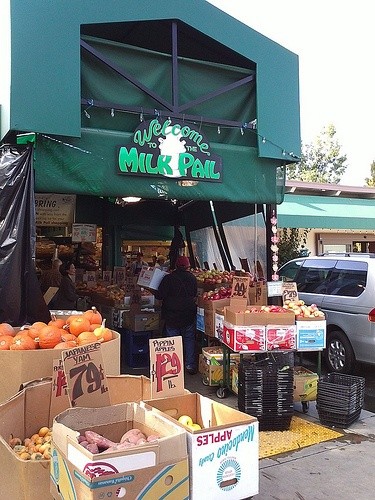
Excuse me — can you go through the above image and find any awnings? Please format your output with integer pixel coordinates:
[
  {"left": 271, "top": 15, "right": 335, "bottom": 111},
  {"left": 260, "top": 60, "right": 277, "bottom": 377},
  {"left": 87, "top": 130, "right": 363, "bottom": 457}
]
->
[{"left": 223, "top": 194, "right": 375, "bottom": 230}]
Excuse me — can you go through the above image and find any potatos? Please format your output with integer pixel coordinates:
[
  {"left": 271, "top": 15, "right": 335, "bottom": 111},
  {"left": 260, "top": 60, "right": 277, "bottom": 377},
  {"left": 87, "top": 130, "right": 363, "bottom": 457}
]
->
[{"left": 77, "top": 428, "right": 159, "bottom": 454}]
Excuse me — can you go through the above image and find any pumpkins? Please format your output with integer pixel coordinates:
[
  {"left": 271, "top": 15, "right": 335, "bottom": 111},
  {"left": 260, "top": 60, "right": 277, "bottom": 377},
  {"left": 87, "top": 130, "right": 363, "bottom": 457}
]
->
[{"left": 0, "top": 306, "right": 112, "bottom": 351}]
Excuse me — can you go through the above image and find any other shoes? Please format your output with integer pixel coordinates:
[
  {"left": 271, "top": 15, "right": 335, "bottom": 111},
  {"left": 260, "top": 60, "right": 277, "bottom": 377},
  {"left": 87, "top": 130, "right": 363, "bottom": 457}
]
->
[{"left": 186, "top": 369, "right": 195, "bottom": 375}]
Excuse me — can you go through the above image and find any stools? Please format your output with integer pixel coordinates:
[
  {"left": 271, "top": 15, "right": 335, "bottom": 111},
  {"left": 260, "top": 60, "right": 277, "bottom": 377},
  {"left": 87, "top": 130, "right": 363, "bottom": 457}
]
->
[{"left": 125, "top": 329, "right": 153, "bottom": 369}]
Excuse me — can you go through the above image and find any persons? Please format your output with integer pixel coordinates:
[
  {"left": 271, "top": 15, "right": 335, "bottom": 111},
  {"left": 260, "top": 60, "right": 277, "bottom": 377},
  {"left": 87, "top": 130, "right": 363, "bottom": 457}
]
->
[
  {"left": 150, "top": 255, "right": 164, "bottom": 267},
  {"left": 57, "top": 262, "right": 78, "bottom": 310},
  {"left": 150, "top": 256, "right": 198, "bottom": 373},
  {"left": 131, "top": 252, "right": 148, "bottom": 274},
  {"left": 39, "top": 258, "right": 63, "bottom": 309}
]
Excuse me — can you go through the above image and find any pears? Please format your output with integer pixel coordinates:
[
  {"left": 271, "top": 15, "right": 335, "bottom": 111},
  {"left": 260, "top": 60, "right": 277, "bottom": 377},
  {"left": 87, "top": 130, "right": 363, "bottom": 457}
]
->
[
  {"left": 111, "top": 291, "right": 125, "bottom": 301},
  {"left": 10, "top": 425, "right": 49, "bottom": 460}
]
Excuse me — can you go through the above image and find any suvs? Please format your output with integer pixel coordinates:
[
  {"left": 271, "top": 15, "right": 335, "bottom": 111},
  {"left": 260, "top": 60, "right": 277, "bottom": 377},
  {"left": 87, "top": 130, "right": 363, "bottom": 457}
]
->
[{"left": 275, "top": 252, "right": 375, "bottom": 374}]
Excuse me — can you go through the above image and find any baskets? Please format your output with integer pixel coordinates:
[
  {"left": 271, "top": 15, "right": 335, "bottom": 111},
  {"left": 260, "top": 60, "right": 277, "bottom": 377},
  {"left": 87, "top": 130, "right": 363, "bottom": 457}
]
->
[
  {"left": 237, "top": 363, "right": 294, "bottom": 431},
  {"left": 316, "top": 372, "right": 365, "bottom": 429}
]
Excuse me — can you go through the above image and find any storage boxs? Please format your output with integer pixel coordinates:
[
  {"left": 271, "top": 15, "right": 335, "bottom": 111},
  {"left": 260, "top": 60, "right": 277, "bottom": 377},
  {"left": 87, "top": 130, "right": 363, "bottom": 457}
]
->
[
  {"left": 0, "top": 310, "right": 260, "bottom": 500},
  {"left": 78, "top": 291, "right": 163, "bottom": 332},
  {"left": 197, "top": 281, "right": 327, "bottom": 401},
  {"left": 136, "top": 265, "right": 170, "bottom": 290}
]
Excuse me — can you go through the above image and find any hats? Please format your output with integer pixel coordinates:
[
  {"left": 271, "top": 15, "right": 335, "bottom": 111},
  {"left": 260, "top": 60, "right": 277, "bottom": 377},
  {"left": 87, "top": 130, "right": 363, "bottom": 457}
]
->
[
  {"left": 176, "top": 256, "right": 190, "bottom": 268},
  {"left": 157, "top": 255, "right": 165, "bottom": 260},
  {"left": 137, "top": 252, "right": 143, "bottom": 257}
]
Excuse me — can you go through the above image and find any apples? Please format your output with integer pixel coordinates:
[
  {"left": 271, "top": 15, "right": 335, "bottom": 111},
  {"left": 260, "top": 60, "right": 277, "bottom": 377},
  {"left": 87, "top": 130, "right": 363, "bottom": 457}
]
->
[
  {"left": 192, "top": 269, "right": 234, "bottom": 300},
  {"left": 178, "top": 415, "right": 202, "bottom": 432},
  {"left": 239, "top": 300, "right": 324, "bottom": 317}
]
